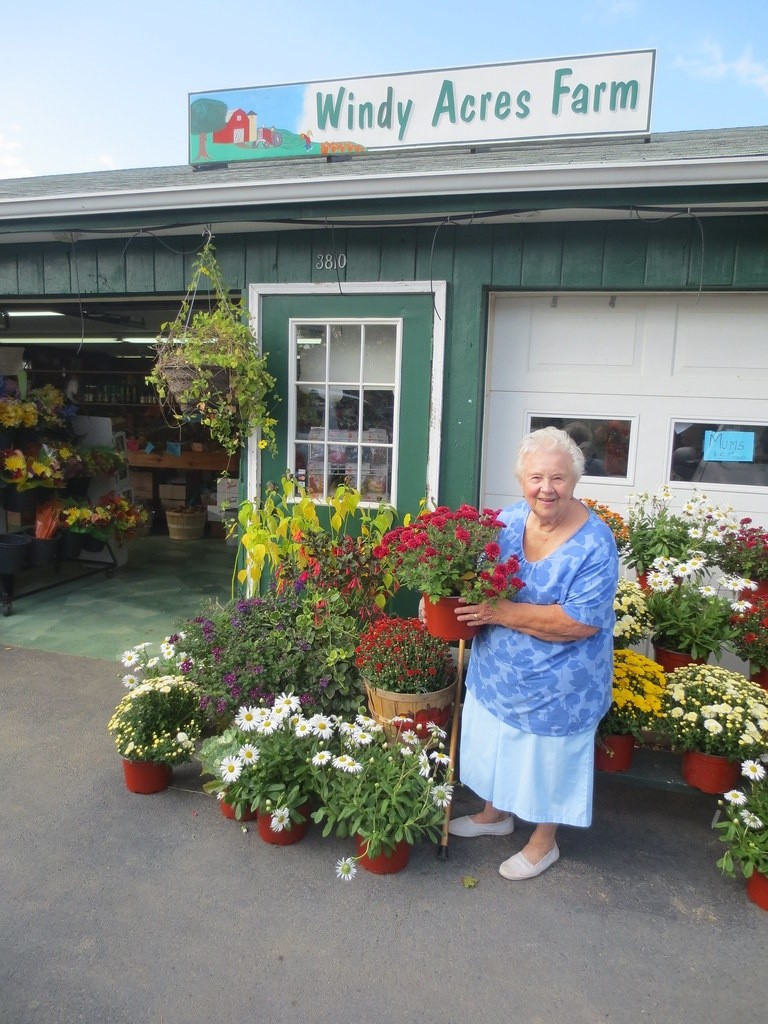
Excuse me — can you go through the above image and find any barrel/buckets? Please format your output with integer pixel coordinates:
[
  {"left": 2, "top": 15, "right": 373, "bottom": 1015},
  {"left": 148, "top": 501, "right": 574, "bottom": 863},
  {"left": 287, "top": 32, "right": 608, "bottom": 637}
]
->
[
  {"left": 0, "top": 534, "right": 33, "bottom": 574},
  {"left": 167, "top": 509, "right": 207, "bottom": 540},
  {"left": 26, "top": 530, "right": 60, "bottom": 566},
  {"left": 367, "top": 669, "right": 461, "bottom": 752}
]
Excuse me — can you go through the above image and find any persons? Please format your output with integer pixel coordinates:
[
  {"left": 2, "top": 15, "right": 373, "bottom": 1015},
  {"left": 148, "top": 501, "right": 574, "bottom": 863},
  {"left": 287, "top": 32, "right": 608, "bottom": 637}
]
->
[{"left": 422, "top": 427, "right": 618, "bottom": 881}]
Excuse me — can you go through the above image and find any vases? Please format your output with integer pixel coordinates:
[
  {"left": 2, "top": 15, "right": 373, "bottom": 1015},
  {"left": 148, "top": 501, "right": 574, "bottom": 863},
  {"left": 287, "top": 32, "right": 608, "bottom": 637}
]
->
[
  {"left": 2, "top": 481, "right": 38, "bottom": 512},
  {"left": 253, "top": 803, "right": 310, "bottom": 847},
  {"left": 123, "top": 758, "right": 173, "bottom": 795},
  {"left": 596, "top": 734, "right": 636, "bottom": 771},
  {"left": 220, "top": 797, "right": 257, "bottom": 822},
  {"left": 355, "top": 833, "right": 408, "bottom": 876},
  {"left": 58, "top": 477, "right": 92, "bottom": 502},
  {"left": 747, "top": 867, "right": 768, "bottom": 911},
  {"left": 748, "top": 659, "right": 768, "bottom": 692},
  {"left": 423, "top": 591, "right": 482, "bottom": 641},
  {"left": 650, "top": 635, "right": 707, "bottom": 674},
  {"left": 167, "top": 509, "right": 207, "bottom": 539},
  {"left": 0, "top": 534, "right": 106, "bottom": 575},
  {"left": 682, "top": 749, "right": 742, "bottom": 794}
]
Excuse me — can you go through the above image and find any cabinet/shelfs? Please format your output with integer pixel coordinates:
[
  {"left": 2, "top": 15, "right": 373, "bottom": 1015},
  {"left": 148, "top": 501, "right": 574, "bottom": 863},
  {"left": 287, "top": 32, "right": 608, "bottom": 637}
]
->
[{"left": 17, "top": 362, "right": 239, "bottom": 472}]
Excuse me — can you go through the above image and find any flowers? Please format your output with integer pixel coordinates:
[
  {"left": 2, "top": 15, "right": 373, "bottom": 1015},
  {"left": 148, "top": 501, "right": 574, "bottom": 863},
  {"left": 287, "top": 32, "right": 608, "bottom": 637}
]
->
[
  {"left": 106, "top": 471, "right": 768, "bottom": 879},
  {"left": 0, "top": 383, "right": 149, "bottom": 540}
]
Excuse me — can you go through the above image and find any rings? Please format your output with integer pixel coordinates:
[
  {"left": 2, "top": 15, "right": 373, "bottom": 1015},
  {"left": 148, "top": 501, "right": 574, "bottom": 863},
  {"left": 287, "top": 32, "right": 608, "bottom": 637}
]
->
[{"left": 475, "top": 613, "right": 480, "bottom": 621}]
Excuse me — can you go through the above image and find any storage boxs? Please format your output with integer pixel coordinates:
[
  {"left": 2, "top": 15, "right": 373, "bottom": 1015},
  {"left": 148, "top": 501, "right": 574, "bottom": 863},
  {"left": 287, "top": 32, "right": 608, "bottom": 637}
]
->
[
  {"left": 158, "top": 484, "right": 187, "bottom": 511},
  {"left": 208, "top": 505, "right": 224, "bottom": 522},
  {"left": 205, "top": 478, "right": 220, "bottom": 510},
  {"left": 216, "top": 477, "right": 238, "bottom": 510},
  {"left": 128, "top": 472, "right": 154, "bottom": 499}
]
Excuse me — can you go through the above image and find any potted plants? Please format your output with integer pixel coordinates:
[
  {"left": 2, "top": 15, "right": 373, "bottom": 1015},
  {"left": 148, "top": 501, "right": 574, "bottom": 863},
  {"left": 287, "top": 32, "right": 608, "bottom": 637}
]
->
[{"left": 145, "top": 232, "right": 281, "bottom": 483}]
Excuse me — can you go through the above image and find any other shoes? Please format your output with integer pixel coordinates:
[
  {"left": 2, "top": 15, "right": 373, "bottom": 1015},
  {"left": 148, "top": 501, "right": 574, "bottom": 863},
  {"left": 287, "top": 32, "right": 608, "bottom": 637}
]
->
[
  {"left": 448, "top": 814, "right": 514, "bottom": 838},
  {"left": 499, "top": 840, "right": 560, "bottom": 880}
]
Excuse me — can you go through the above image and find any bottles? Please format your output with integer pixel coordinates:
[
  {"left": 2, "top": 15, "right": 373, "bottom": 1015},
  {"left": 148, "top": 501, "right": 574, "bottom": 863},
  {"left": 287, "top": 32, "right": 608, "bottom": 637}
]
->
[{"left": 83, "top": 381, "right": 156, "bottom": 404}]
